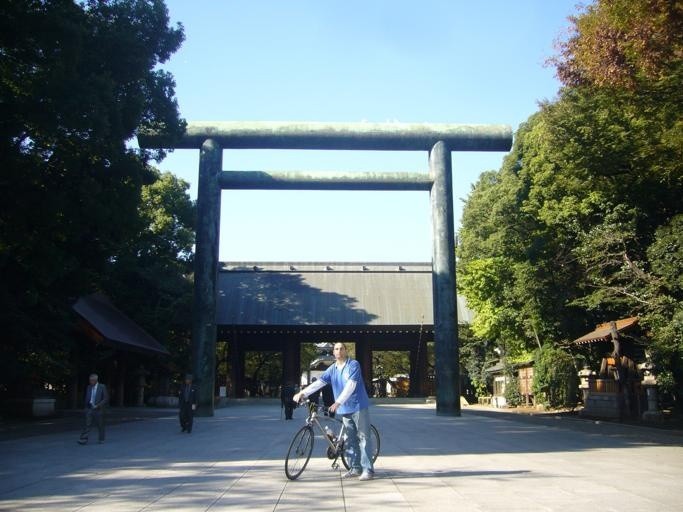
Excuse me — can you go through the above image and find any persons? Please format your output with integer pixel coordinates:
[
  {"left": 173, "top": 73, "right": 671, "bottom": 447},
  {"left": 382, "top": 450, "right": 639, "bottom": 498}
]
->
[
  {"left": 269, "top": 376, "right": 335, "bottom": 421},
  {"left": 291, "top": 341, "right": 375, "bottom": 481},
  {"left": 76, "top": 373, "right": 109, "bottom": 445},
  {"left": 175, "top": 374, "right": 196, "bottom": 435}
]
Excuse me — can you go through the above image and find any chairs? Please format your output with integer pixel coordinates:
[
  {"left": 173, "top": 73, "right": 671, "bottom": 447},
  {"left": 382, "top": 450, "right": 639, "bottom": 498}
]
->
[
  {"left": 339, "top": 468, "right": 362, "bottom": 479},
  {"left": 77, "top": 440, "right": 106, "bottom": 445},
  {"left": 358, "top": 469, "right": 374, "bottom": 480}
]
